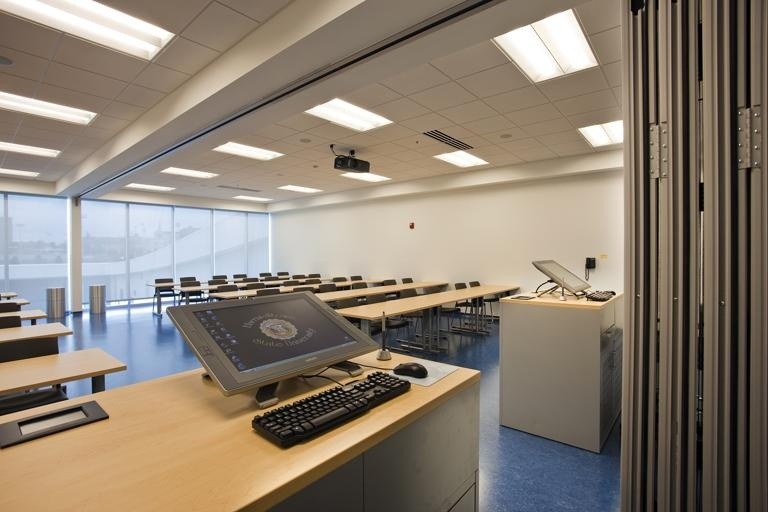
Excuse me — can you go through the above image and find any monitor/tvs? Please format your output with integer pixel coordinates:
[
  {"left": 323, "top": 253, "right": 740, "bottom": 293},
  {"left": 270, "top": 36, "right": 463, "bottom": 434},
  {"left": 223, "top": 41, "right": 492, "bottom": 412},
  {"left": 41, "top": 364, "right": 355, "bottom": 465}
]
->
[
  {"left": 532, "top": 260, "right": 591, "bottom": 301},
  {"left": 166, "top": 291, "right": 381, "bottom": 408}
]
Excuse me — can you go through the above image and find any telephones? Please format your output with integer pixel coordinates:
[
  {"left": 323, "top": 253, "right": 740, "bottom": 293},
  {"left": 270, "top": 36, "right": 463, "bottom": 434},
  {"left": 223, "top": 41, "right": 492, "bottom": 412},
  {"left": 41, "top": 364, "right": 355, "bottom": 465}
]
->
[{"left": 586, "top": 258, "right": 595, "bottom": 268}]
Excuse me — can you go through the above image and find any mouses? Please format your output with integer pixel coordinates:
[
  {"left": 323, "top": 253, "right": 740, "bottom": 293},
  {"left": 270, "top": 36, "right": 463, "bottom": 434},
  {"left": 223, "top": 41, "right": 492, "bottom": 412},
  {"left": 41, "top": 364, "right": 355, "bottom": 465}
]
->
[
  {"left": 393, "top": 362, "right": 428, "bottom": 378},
  {"left": 610, "top": 291, "right": 616, "bottom": 295}
]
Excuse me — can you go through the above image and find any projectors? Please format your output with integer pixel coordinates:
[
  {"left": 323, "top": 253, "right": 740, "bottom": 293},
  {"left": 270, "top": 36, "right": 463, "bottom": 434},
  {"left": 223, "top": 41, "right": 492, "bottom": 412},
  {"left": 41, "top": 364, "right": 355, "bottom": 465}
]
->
[{"left": 334, "top": 157, "right": 370, "bottom": 173}]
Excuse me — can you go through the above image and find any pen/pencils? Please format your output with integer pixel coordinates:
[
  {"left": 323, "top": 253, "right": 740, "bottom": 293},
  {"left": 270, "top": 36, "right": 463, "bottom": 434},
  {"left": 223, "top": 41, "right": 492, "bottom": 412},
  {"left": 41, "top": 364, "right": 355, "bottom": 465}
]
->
[
  {"left": 382, "top": 311, "right": 385, "bottom": 351},
  {"left": 562, "top": 277, "right": 564, "bottom": 296}
]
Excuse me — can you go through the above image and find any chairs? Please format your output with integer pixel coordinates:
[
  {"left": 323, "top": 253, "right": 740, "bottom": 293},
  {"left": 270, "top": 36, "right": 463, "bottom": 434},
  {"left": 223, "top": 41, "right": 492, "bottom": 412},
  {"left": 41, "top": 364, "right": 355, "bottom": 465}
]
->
[
  {"left": 212, "top": 275, "right": 228, "bottom": 280},
  {"left": 177, "top": 277, "right": 196, "bottom": 305},
  {"left": 352, "top": 283, "right": 368, "bottom": 288},
  {"left": 306, "top": 279, "right": 321, "bottom": 284},
  {"left": 450, "top": 283, "right": 486, "bottom": 336},
  {"left": 365, "top": 293, "right": 411, "bottom": 355},
  {"left": 294, "top": 287, "right": 315, "bottom": 294},
  {"left": 181, "top": 281, "right": 205, "bottom": 305},
  {"left": 257, "top": 288, "right": 280, "bottom": 296},
  {"left": 277, "top": 272, "right": 289, "bottom": 276},
  {"left": 397, "top": 288, "right": 440, "bottom": 350},
  {"left": 309, "top": 274, "right": 321, "bottom": 278},
  {"left": 401, "top": 277, "right": 414, "bottom": 283},
  {"left": 259, "top": 273, "right": 271, "bottom": 277},
  {"left": 233, "top": 274, "right": 247, "bottom": 278},
  {"left": 336, "top": 297, "right": 362, "bottom": 331},
  {"left": 247, "top": 283, "right": 264, "bottom": 289},
  {"left": 384, "top": 279, "right": 396, "bottom": 285},
  {"left": 293, "top": 275, "right": 305, "bottom": 278},
  {"left": 152, "top": 279, "right": 176, "bottom": 312},
  {"left": 284, "top": 281, "right": 299, "bottom": 285},
  {"left": 423, "top": 284, "right": 463, "bottom": 339},
  {"left": 319, "top": 284, "right": 336, "bottom": 293},
  {"left": 350, "top": 275, "right": 363, "bottom": 280},
  {"left": 332, "top": 277, "right": 347, "bottom": 283},
  {"left": 243, "top": 278, "right": 258, "bottom": 281},
  {"left": 469, "top": 281, "right": 499, "bottom": 324},
  {"left": 218, "top": 285, "right": 240, "bottom": 301},
  {"left": 265, "top": 277, "right": 279, "bottom": 281},
  {"left": 207, "top": 280, "right": 225, "bottom": 303}
]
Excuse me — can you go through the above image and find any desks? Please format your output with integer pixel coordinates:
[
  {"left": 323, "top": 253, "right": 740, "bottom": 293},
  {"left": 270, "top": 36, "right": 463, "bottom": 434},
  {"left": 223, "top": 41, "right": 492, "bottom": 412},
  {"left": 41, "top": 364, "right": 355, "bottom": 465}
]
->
[
  {"left": 0, "top": 292, "right": 128, "bottom": 420},
  {"left": 313, "top": 281, "right": 448, "bottom": 307},
  {"left": 145, "top": 275, "right": 292, "bottom": 318},
  {"left": 335, "top": 281, "right": 519, "bottom": 358},
  {"left": 209, "top": 280, "right": 355, "bottom": 299}
]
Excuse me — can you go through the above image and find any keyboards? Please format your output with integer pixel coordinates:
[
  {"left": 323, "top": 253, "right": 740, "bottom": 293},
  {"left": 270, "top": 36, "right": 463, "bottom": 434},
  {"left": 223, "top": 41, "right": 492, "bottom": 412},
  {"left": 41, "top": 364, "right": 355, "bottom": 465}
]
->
[
  {"left": 586, "top": 291, "right": 613, "bottom": 301},
  {"left": 252, "top": 371, "right": 411, "bottom": 449}
]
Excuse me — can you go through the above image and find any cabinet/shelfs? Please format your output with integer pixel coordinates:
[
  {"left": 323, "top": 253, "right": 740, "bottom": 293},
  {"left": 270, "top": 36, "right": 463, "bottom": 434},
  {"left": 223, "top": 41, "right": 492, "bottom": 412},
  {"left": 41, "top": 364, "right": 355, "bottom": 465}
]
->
[
  {"left": 0, "top": 338, "right": 483, "bottom": 512},
  {"left": 496, "top": 278, "right": 624, "bottom": 458}
]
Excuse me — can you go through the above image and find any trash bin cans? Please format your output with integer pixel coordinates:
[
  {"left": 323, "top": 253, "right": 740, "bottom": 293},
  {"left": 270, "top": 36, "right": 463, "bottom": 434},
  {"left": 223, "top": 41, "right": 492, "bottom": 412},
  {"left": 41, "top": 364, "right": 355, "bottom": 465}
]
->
[
  {"left": 47, "top": 288, "right": 65, "bottom": 319},
  {"left": 89, "top": 286, "right": 106, "bottom": 314}
]
